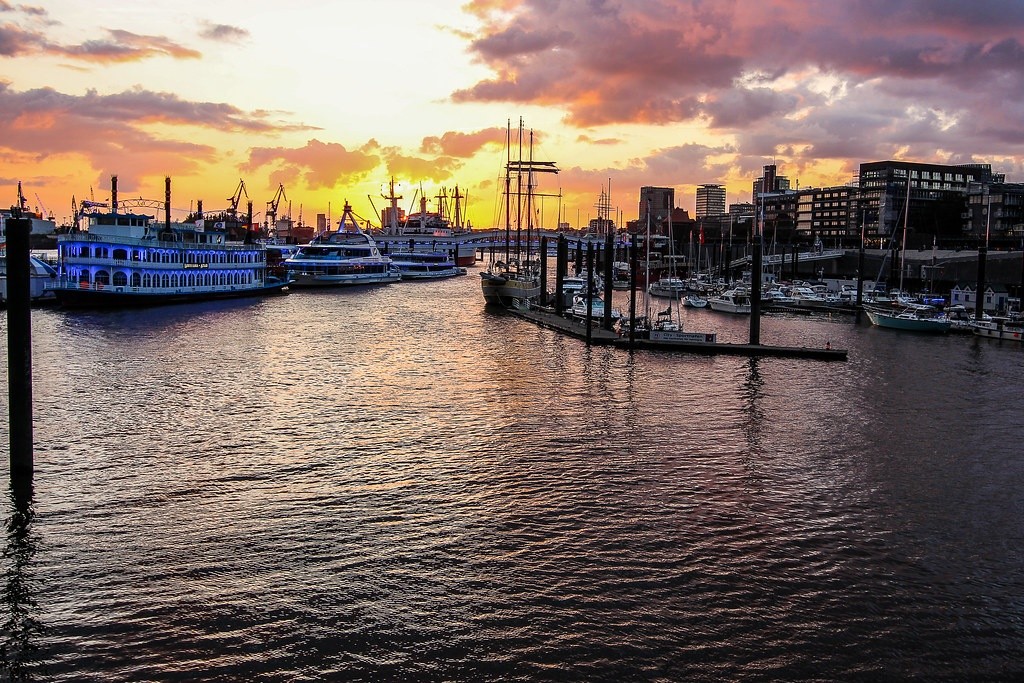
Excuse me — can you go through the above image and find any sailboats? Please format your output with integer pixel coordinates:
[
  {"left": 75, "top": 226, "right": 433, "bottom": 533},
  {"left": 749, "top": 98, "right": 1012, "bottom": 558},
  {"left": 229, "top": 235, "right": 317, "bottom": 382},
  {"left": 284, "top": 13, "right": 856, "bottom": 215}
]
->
[
  {"left": 478, "top": 115, "right": 1024, "bottom": 363},
  {"left": 0, "top": 174, "right": 481, "bottom": 309}
]
